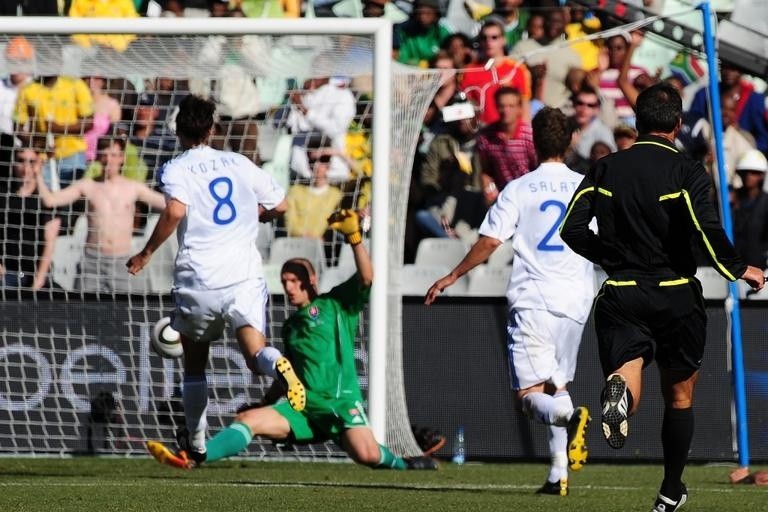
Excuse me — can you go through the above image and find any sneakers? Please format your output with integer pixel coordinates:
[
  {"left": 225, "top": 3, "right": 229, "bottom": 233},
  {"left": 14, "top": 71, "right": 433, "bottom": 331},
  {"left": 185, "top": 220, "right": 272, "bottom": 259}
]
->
[
  {"left": 147, "top": 430, "right": 206, "bottom": 470},
  {"left": 275, "top": 357, "right": 306, "bottom": 412},
  {"left": 534, "top": 480, "right": 569, "bottom": 496},
  {"left": 650, "top": 482, "right": 686, "bottom": 512},
  {"left": 402, "top": 457, "right": 438, "bottom": 471},
  {"left": 601, "top": 374, "right": 627, "bottom": 449},
  {"left": 566, "top": 407, "right": 591, "bottom": 471}
]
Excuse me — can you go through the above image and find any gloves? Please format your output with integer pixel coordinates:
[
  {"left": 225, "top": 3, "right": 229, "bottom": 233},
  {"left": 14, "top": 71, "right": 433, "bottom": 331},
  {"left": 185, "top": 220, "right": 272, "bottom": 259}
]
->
[{"left": 328, "top": 209, "right": 360, "bottom": 244}]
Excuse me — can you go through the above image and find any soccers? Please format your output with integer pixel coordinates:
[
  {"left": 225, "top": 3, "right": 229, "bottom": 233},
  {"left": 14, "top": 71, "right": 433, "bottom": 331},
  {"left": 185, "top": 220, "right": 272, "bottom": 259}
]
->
[{"left": 150, "top": 316, "right": 185, "bottom": 359}]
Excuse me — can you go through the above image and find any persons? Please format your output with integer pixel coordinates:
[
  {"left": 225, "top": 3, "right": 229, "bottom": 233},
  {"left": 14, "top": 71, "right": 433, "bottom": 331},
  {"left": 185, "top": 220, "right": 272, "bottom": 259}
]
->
[
  {"left": 147, "top": 209, "right": 441, "bottom": 471},
  {"left": 424, "top": 106, "right": 600, "bottom": 496},
  {"left": 558, "top": 82, "right": 764, "bottom": 512},
  {"left": 1, "top": 0, "right": 767, "bottom": 301},
  {"left": 124, "top": 95, "right": 307, "bottom": 462}
]
